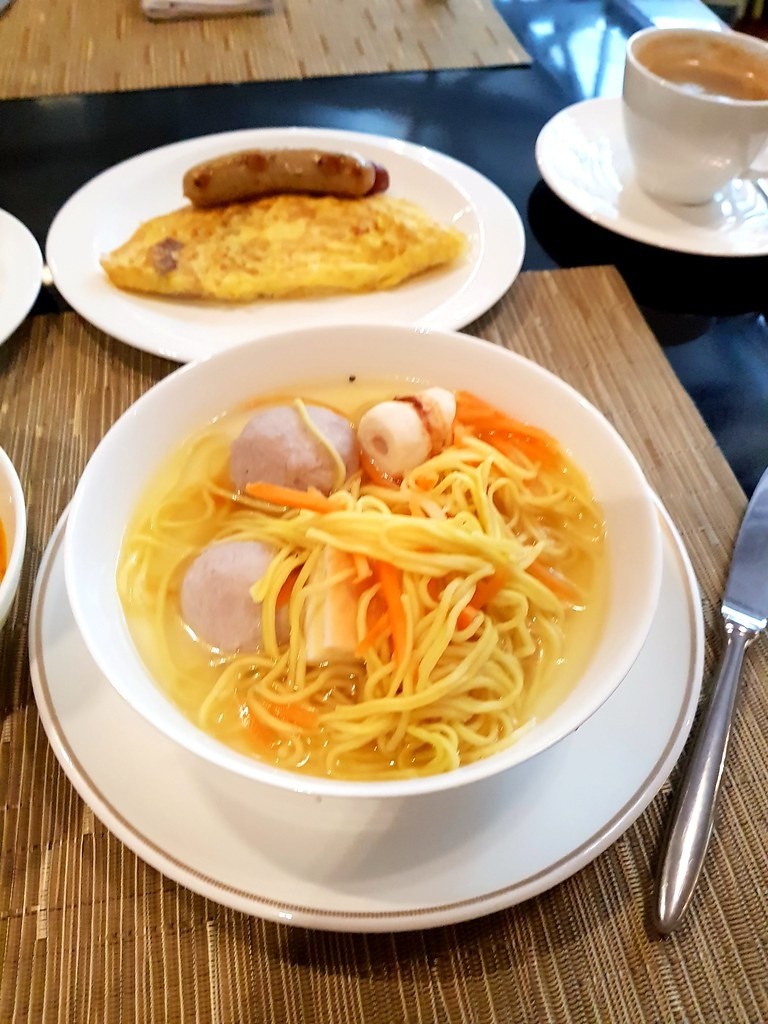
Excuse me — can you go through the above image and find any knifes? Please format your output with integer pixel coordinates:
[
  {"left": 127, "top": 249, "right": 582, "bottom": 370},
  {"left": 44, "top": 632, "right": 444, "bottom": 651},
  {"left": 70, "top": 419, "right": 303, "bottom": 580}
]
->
[{"left": 653, "top": 466, "right": 768, "bottom": 934}]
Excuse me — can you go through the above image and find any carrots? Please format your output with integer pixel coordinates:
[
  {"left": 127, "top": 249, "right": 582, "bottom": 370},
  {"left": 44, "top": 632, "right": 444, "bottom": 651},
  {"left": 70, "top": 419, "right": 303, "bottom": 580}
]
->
[{"left": 242, "top": 393, "right": 587, "bottom": 740}]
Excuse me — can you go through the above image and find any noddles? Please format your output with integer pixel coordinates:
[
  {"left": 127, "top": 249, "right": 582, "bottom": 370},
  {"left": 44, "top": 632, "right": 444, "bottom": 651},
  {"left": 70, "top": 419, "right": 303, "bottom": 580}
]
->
[{"left": 118, "top": 376, "right": 611, "bottom": 783}]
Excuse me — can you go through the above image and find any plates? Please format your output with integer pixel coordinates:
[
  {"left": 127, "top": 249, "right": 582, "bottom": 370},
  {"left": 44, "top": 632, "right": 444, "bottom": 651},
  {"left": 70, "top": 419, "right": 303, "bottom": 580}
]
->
[
  {"left": 27, "top": 495, "right": 704, "bottom": 934},
  {"left": 0, "top": 209, "right": 44, "bottom": 346},
  {"left": 534, "top": 97, "right": 768, "bottom": 257},
  {"left": 46, "top": 126, "right": 527, "bottom": 363}
]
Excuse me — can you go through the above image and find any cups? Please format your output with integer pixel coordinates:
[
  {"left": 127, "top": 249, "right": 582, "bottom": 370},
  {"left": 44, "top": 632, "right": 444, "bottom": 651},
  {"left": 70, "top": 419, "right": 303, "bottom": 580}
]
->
[{"left": 621, "top": 26, "right": 768, "bottom": 204}]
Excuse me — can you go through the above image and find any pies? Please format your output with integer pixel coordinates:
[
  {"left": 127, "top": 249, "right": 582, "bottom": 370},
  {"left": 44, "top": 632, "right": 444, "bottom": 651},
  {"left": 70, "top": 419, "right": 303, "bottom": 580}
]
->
[{"left": 98, "top": 190, "right": 467, "bottom": 302}]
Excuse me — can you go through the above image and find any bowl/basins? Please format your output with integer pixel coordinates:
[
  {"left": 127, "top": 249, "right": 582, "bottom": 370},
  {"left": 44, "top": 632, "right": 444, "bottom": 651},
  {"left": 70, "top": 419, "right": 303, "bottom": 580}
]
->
[
  {"left": 62, "top": 324, "right": 664, "bottom": 796},
  {"left": 0, "top": 446, "right": 28, "bottom": 630}
]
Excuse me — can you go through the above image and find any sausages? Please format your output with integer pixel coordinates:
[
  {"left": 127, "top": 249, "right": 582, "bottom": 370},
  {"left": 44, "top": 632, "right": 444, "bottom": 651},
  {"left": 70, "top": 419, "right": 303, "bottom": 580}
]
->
[{"left": 181, "top": 144, "right": 389, "bottom": 206}]
